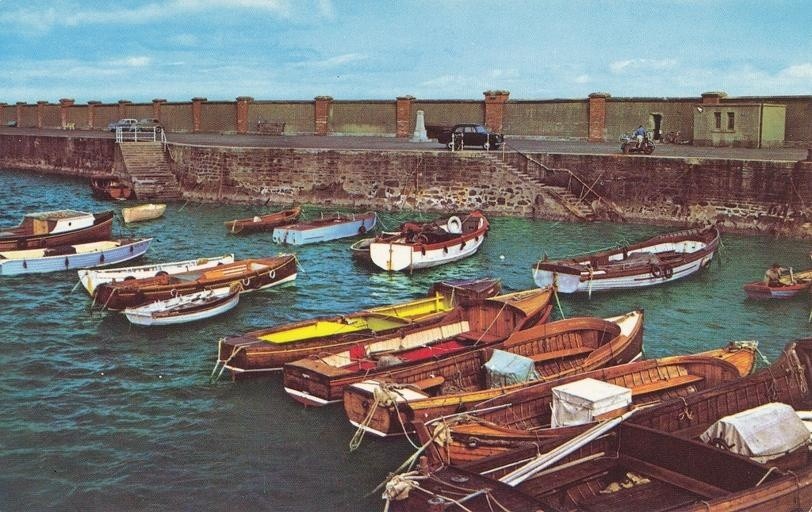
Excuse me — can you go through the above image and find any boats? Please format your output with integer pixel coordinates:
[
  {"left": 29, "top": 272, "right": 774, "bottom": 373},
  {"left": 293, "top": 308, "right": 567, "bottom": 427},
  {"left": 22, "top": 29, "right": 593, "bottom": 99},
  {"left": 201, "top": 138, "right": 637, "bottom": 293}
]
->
[{"left": 87, "top": 162, "right": 138, "bottom": 205}]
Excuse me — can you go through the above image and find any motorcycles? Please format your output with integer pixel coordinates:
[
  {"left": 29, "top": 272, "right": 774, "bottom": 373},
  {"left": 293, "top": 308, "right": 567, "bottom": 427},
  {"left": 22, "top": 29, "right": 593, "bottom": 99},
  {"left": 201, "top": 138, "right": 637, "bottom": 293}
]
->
[{"left": 621, "top": 131, "right": 658, "bottom": 157}]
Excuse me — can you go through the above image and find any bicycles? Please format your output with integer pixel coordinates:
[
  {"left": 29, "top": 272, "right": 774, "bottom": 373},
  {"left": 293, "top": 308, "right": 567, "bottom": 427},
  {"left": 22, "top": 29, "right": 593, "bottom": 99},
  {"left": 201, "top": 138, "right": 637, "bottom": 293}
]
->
[{"left": 661, "top": 131, "right": 682, "bottom": 145}]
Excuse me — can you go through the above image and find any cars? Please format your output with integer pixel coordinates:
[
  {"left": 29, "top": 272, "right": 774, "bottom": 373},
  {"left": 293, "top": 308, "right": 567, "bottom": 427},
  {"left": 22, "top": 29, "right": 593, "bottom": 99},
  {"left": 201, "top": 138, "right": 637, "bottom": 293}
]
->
[
  {"left": 435, "top": 122, "right": 507, "bottom": 154},
  {"left": 129, "top": 117, "right": 168, "bottom": 135},
  {"left": 106, "top": 116, "right": 140, "bottom": 134}
]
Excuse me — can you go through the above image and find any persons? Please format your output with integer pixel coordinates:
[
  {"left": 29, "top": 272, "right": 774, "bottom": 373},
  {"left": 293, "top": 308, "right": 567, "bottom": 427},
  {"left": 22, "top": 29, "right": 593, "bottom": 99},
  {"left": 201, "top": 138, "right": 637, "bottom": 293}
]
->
[
  {"left": 762, "top": 263, "right": 789, "bottom": 287},
  {"left": 635, "top": 125, "right": 647, "bottom": 148}
]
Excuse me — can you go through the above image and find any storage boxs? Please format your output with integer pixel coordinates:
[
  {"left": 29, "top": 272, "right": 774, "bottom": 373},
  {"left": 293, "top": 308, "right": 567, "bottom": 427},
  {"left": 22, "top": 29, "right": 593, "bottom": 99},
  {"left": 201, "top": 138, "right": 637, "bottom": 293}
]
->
[{"left": 550, "top": 378, "right": 633, "bottom": 430}]
